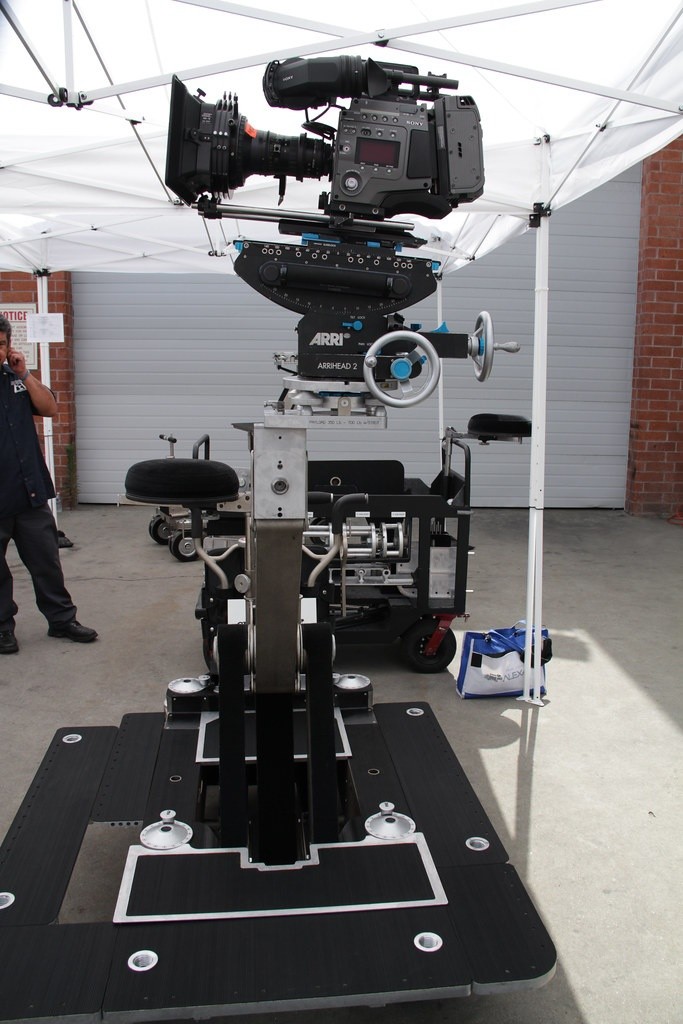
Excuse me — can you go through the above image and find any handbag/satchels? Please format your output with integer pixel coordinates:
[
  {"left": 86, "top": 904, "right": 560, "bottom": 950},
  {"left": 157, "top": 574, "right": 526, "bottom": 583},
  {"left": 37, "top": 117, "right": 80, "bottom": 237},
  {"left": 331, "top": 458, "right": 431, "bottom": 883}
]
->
[{"left": 455, "top": 620, "right": 553, "bottom": 699}]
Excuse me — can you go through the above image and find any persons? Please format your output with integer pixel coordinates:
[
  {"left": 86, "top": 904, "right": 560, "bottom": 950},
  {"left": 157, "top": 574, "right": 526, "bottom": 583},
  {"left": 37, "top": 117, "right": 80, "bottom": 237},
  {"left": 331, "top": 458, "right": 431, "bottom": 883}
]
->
[{"left": 0, "top": 314, "right": 97, "bottom": 652}]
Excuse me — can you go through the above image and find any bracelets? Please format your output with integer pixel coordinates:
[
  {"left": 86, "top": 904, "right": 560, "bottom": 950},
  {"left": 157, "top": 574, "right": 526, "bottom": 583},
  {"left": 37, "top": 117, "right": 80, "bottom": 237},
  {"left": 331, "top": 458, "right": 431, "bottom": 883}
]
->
[{"left": 22, "top": 369, "right": 30, "bottom": 380}]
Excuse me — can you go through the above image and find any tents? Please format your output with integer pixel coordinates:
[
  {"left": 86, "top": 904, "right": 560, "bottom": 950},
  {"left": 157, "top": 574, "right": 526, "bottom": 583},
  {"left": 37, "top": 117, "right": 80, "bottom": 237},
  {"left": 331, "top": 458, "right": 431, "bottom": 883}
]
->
[{"left": 0, "top": 0, "right": 683, "bottom": 702}]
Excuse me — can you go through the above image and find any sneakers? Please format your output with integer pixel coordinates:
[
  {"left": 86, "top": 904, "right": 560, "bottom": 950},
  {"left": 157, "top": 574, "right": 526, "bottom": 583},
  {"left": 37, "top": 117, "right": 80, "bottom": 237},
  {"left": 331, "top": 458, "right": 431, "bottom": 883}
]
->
[
  {"left": 0, "top": 630, "right": 18, "bottom": 652},
  {"left": 47, "top": 619, "right": 98, "bottom": 642}
]
